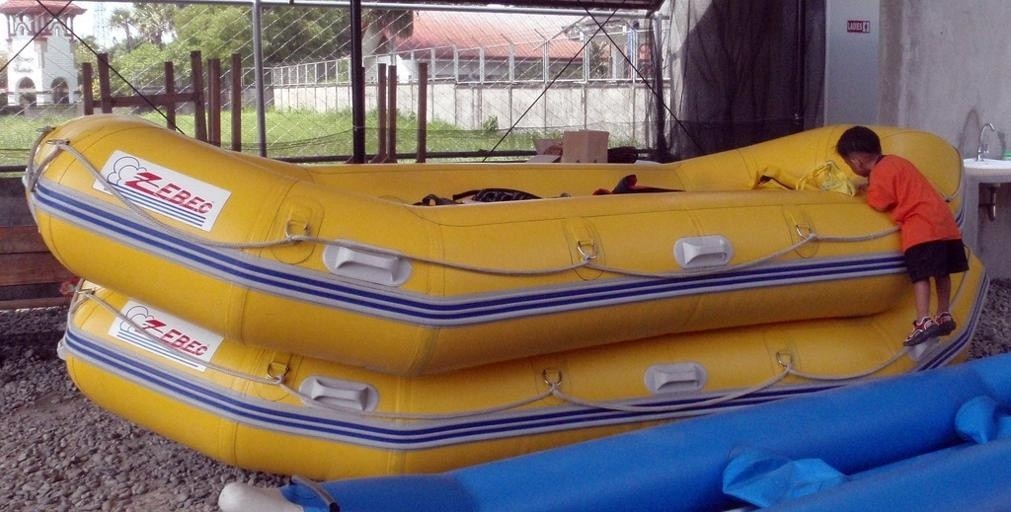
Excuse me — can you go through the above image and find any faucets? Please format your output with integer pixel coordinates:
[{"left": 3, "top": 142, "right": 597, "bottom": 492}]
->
[{"left": 976, "top": 122, "right": 997, "bottom": 161}]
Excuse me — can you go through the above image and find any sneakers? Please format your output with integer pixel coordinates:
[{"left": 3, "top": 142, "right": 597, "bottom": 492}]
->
[{"left": 902, "top": 311, "right": 957, "bottom": 346}]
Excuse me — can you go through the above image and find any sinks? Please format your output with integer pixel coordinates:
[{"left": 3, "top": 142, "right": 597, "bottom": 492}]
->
[{"left": 962, "top": 157, "right": 1011, "bottom": 184}]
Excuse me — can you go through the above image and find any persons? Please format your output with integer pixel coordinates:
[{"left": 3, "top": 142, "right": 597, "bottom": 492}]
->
[{"left": 836, "top": 126, "right": 968, "bottom": 346}]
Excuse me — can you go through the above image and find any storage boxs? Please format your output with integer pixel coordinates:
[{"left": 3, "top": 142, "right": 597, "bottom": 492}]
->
[{"left": 562, "top": 129, "right": 609, "bottom": 164}]
